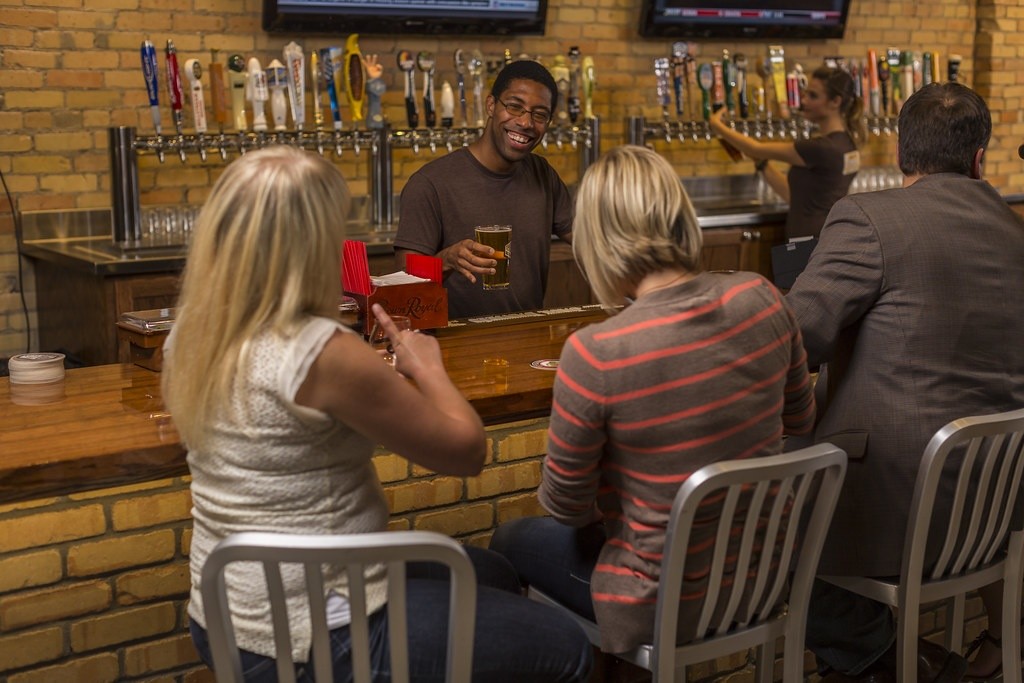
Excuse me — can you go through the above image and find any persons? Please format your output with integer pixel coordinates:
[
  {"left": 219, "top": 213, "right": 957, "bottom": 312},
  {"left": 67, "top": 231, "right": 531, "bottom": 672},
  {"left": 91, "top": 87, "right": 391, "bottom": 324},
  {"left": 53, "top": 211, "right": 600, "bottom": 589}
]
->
[
  {"left": 777, "top": 78, "right": 1024, "bottom": 683},
  {"left": 482, "top": 141, "right": 818, "bottom": 656},
  {"left": 161, "top": 146, "right": 594, "bottom": 682},
  {"left": 707, "top": 68, "right": 863, "bottom": 295},
  {"left": 388, "top": 59, "right": 578, "bottom": 318}
]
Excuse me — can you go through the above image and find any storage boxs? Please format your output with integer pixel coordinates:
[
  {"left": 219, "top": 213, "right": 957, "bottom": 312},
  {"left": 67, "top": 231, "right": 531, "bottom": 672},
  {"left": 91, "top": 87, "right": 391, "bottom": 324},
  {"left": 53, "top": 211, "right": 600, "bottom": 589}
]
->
[{"left": 346, "top": 283, "right": 449, "bottom": 338}]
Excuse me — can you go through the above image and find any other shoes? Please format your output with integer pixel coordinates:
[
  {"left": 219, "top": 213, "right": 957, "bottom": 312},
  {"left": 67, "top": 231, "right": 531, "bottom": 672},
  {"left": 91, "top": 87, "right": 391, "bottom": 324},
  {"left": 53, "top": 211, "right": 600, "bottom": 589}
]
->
[{"left": 963, "top": 630, "right": 1004, "bottom": 682}]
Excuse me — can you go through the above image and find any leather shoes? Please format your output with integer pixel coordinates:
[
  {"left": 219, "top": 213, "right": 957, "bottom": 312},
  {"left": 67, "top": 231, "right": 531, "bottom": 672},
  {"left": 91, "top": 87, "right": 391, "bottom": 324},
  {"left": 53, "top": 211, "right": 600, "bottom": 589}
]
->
[{"left": 819, "top": 636, "right": 969, "bottom": 683}]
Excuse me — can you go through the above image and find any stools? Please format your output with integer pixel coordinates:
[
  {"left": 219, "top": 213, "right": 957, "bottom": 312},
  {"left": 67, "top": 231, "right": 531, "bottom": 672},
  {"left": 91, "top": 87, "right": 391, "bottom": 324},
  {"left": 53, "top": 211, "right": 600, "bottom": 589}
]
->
[
  {"left": 528, "top": 443, "right": 848, "bottom": 682},
  {"left": 818, "top": 408, "right": 1024, "bottom": 682},
  {"left": 203, "top": 533, "right": 473, "bottom": 682}
]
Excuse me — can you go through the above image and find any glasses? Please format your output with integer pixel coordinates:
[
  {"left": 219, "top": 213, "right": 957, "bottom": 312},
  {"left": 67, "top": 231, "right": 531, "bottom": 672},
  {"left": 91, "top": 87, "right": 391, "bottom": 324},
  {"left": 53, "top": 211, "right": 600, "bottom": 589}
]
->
[{"left": 495, "top": 94, "right": 550, "bottom": 124}]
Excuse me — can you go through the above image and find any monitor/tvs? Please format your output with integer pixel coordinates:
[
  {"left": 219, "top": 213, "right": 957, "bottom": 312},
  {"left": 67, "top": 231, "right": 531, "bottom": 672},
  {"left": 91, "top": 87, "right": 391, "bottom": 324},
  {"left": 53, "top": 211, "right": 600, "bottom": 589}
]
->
[
  {"left": 638, "top": 0, "right": 851, "bottom": 39},
  {"left": 261, "top": 0, "right": 548, "bottom": 38}
]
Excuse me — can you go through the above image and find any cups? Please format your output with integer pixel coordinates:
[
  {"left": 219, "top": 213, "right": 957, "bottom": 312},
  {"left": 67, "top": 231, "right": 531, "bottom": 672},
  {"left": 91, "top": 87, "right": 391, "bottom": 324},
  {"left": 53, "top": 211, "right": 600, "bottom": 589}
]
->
[
  {"left": 370, "top": 316, "right": 411, "bottom": 369},
  {"left": 140, "top": 203, "right": 195, "bottom": 239},
  {"left": 475, "top": 224, "right": 514, "bottom": 291}
]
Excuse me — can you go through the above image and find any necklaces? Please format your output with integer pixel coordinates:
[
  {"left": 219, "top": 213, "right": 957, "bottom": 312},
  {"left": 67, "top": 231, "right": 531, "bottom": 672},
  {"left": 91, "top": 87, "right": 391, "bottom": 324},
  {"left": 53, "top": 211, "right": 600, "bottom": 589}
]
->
[{"left": 637, "top": 267, "right": 690, "bottom": 296}]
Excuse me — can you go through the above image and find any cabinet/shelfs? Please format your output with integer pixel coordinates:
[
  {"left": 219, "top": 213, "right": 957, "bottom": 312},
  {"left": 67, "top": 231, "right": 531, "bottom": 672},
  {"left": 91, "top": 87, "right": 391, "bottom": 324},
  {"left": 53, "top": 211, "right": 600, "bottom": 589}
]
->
[
  {"left": 384, "top": 117, "right": 598, "bottom": 246},
  {"left": 30, "top": 229, "right": 786, "bottom": 365},
  {"left": 767, "top": 114, "right": 905, "bottom": 207},
  {"left": 107, "top": 125, "right": 379, "bottom": 251},
  {"left": 627, "top": 113, "right": 765, "bottom": 214}
]
